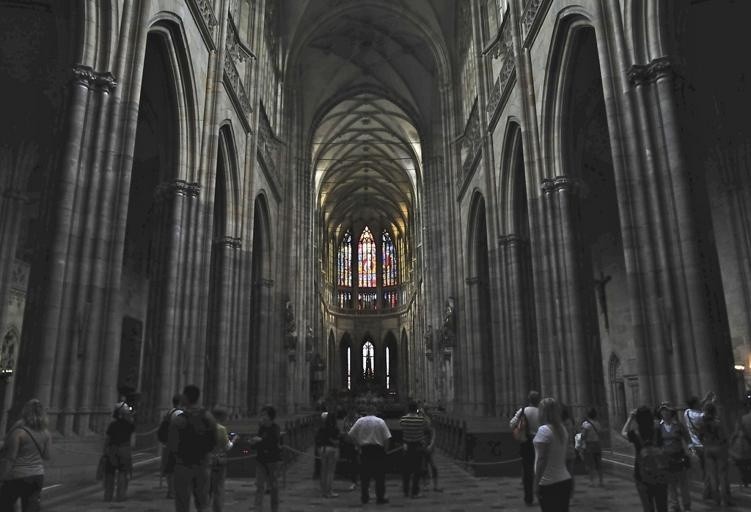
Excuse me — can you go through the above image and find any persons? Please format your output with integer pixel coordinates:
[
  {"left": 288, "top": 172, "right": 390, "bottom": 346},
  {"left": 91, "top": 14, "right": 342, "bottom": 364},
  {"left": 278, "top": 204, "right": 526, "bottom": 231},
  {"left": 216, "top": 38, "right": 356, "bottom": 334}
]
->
[
  {"left": 103, "top": 401, "right": 136, "bottom": 501},
  {"left": 316, "top": 391, "right": 443, "bottom": 503},
  {"left": 532, "top": 397, "right": 572, "bottom": 512},
  {"left": 508, "top": 391, "right": 543, "bottom": 506},
  {"left": 562, "top": 402, "right": 576, "bottom": 497},
  {"left": 622, "top": 390, "right": 750, "bottom": 512},
  {"left": 209, "top": 408, "right": 240, "bottom": 512},
  {"left": 246, "top": 406, "right": 282, "bottom": 511},
  {"left": 159, "top": 394, "right": 184, "bottom": 499},
  {"left": 167, "top": 385, "right": 218, "bottom": 511},
  {"left": 0, "top": 397, "right": 52, "bottom": 511},
  {"left": 580, "top": 408, "right": 606, "bottom": 488}
]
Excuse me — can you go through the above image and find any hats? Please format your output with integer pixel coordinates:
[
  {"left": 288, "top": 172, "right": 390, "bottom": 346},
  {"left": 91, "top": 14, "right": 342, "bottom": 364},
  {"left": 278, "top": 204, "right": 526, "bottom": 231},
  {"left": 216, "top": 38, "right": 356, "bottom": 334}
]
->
[{"left": 657, "top": 401, "right": 675, "bottom": 413}]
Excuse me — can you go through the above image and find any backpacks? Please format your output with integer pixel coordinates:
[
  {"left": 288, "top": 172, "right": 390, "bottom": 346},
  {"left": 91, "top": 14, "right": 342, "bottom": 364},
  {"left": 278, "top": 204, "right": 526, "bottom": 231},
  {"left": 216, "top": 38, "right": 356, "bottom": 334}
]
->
[
  {"left": 158, "top": 408, "right": 180, "bottom": 443},
  {"left": 633, "top": 429, "right": 668, "bottom": 485}
]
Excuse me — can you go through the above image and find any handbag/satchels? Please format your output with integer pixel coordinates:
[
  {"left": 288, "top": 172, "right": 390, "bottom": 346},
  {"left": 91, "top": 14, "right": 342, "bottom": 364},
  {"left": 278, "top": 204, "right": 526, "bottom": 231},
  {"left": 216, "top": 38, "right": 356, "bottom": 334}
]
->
[{"left": 512, "top": 412, "right": 529, "bottom": 445}]
